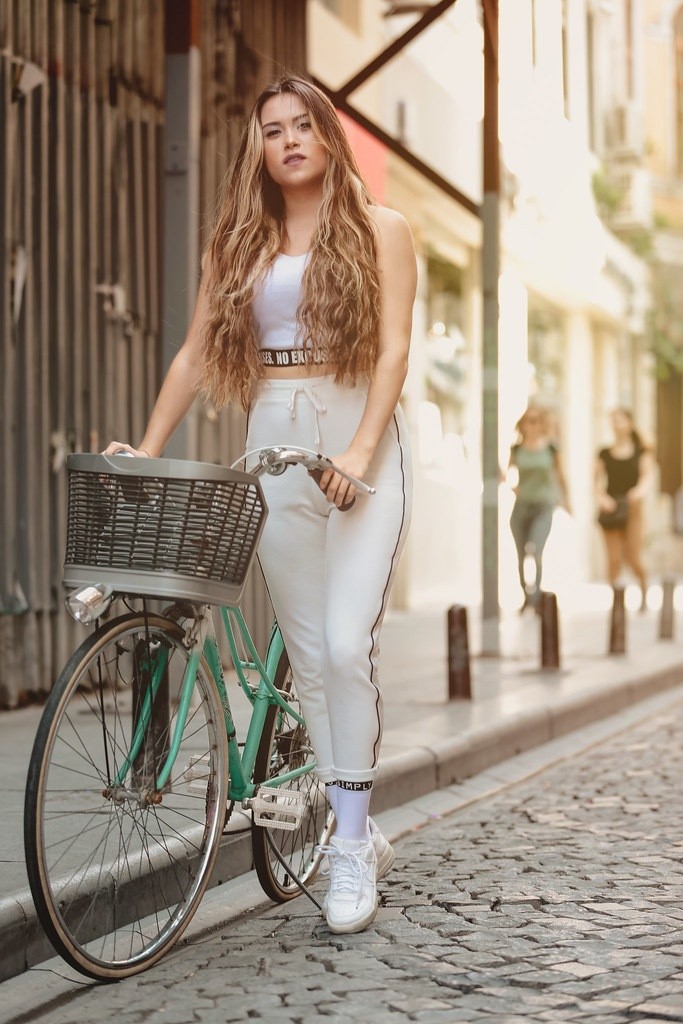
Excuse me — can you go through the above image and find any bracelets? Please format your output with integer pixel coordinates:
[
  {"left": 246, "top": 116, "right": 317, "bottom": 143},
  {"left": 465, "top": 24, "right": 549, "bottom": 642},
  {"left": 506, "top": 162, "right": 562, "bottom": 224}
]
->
[{"left": 138, "top": 449, "right": 150, "bottom": 458}]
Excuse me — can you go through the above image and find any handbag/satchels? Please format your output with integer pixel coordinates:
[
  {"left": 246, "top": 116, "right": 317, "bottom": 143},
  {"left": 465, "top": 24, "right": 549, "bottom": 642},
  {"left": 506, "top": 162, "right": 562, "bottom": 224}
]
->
[{"left": 598, "top": 494, "right": 628, "bottom": 530}]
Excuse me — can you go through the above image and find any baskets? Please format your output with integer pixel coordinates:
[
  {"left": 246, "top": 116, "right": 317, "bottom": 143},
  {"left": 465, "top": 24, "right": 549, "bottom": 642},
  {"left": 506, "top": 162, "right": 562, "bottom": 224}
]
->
[{"left": 62, "top": 453, "right": 269, "bottom": 608}]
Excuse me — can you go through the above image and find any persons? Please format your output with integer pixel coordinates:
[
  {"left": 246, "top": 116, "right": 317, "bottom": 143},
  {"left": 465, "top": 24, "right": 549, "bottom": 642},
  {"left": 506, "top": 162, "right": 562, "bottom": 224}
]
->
[
  {"left": 99, "top": 76, "right": 418, "bottom": 934},
  {"left": 507, "top": 402, "right": 654, "bottom": 618}
]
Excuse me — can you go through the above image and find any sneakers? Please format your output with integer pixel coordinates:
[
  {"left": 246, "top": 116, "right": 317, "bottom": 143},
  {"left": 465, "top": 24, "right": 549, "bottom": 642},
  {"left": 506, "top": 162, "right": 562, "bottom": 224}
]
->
[
  {"left": 316, "top": 834, "right": 378, "bottom": 934},
  {"left": 368, "top": 816, "right": 395, "bottom": 882}
]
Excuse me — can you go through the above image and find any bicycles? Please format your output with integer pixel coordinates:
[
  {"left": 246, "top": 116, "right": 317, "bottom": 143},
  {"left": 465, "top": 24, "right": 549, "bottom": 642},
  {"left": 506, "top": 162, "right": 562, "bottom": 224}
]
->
[{"left": 21, "top": 444, "right": 336, "bottom": 979}]
